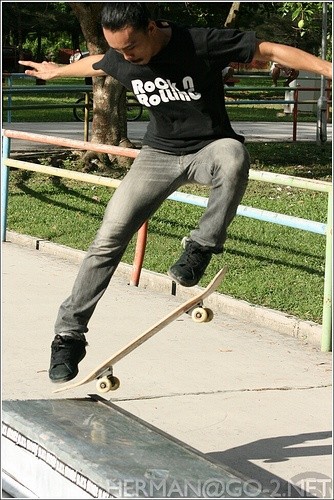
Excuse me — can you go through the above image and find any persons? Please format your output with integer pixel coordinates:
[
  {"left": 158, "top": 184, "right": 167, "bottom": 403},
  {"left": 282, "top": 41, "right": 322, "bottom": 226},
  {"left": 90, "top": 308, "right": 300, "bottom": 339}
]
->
[
  {"left": 221, "top": 62, "right": 239, "bottom": 87},
  {"left": 17, "top": 2, "right": 333, "bottom": 383},
  {"left": 281, "top": 66, "right": 299, "bottom": 114}
]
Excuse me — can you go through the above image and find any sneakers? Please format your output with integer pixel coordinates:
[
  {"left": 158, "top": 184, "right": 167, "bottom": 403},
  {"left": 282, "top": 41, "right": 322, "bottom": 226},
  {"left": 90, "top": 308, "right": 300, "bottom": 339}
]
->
[
  {"left": 48, "top": 332, "right": 85, "bottom": 382},
  {"left": 166, "top": 240, "right": 212, "bottom": 287}
]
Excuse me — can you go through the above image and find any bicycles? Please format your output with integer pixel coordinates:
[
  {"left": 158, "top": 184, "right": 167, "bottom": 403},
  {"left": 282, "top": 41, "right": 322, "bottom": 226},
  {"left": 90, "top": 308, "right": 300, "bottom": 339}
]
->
[{"left": 72, "top": 93, "right": 142, "bottom": 121}]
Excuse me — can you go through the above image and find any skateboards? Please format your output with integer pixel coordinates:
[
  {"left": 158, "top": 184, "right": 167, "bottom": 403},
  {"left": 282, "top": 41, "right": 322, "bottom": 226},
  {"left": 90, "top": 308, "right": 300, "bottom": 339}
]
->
[{"left": 53, "top": 265, "right": 228, "bottom": 394}]
